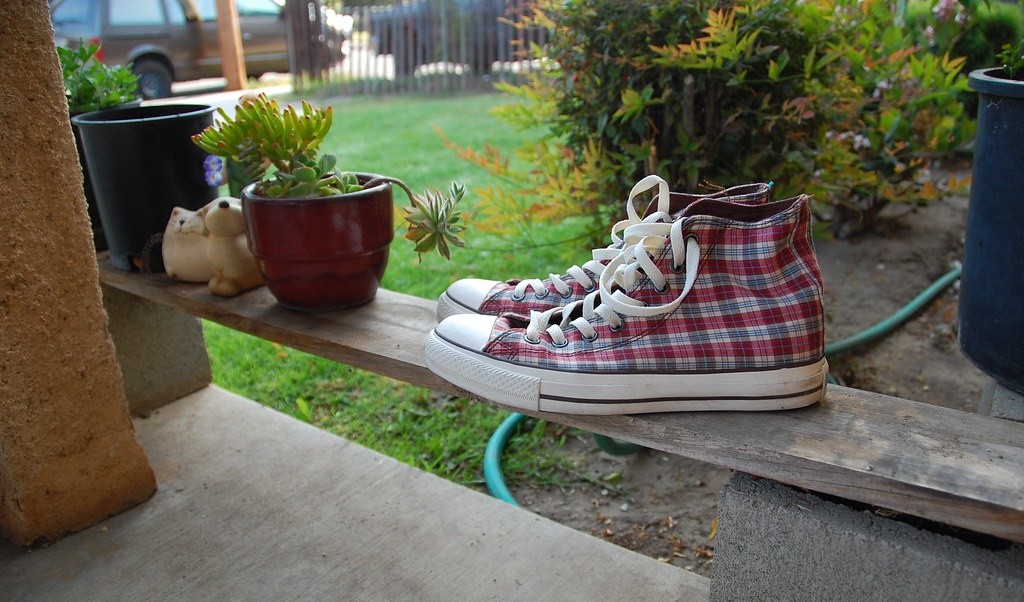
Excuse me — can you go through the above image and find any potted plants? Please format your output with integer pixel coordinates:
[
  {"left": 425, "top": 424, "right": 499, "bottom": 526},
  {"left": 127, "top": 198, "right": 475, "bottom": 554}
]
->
[
  {"left": 56, "top": 35, "right": 143, "bottom": 253},
  {"left": 195, "top": 92, "right": 471, "bottom": 311},
  {"left": 435, "top": 0, "right": 975, "bottom": 244}
]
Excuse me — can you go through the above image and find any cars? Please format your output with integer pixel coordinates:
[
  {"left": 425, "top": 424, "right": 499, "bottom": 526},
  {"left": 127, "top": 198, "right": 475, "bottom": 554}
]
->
[{"left": 52, "top": 1, "right": 350, "bottom": 100}]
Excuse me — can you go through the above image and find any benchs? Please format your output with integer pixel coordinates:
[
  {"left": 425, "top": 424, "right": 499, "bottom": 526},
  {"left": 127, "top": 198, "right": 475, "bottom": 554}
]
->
[{"left": 99, "top": 272, "right": 1024, "bottom": 602}]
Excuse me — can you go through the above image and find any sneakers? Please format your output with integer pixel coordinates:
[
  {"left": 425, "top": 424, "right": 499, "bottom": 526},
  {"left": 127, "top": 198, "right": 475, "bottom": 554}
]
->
[
  {"left": 436, "top": 174, "right": 770, "bottom": 328},
  {"left": 423, "top": 194, "right": 830, "bottom": 415}
]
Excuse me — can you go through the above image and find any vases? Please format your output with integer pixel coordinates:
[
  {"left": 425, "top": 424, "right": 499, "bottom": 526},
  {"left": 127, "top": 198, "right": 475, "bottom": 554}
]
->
[
  {"left": 956, "top": 39, "right": 1024, "bottom": 398},
  {"left": 70, "top": 103, "right": 220, "bottom": 273}
]
